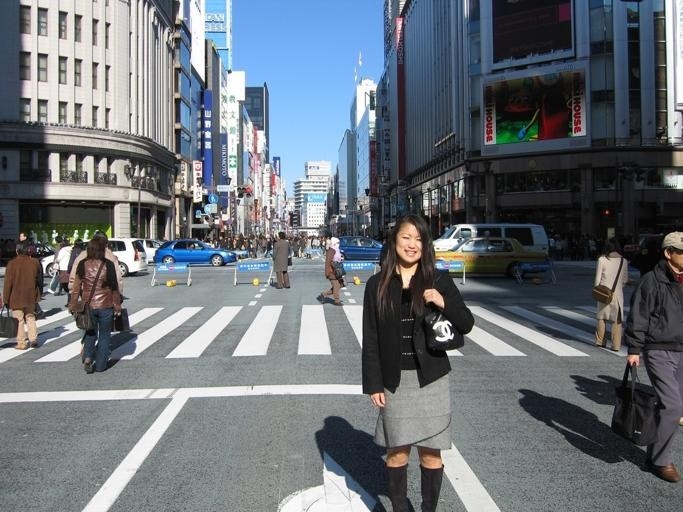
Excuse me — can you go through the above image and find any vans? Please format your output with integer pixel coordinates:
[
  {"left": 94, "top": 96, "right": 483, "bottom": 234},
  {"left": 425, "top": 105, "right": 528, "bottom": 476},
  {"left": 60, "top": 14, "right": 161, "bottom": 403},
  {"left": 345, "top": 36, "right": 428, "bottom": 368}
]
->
[
  {"left": 430, "top": 223, "right": 551, "bottom": 260},
  {"left": 335, "top": 235, "right": 383, "bottom": 263}
]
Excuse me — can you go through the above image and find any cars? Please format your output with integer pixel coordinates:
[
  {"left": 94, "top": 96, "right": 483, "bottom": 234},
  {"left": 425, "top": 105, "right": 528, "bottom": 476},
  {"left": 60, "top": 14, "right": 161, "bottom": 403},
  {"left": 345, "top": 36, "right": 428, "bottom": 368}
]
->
[
  {"left": 0, "top": 236, "right": 254, "bottom": 283},
  {"left": 624, "top": 233, "right": 663, "bottom": 258},
  {"left": 435, "top": 236, "right": 552, "bottom": 282}
]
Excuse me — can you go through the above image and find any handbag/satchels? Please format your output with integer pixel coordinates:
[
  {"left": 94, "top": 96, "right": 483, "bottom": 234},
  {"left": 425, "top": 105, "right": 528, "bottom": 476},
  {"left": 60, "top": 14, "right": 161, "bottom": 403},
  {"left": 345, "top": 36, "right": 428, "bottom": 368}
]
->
[
  {"left": 58, "top": 271, "right": 70, "bottom": 284},
  {"left": 330, "top": 261, "right": 346, "bottom": 280},
  {"left": 115, "top": 309, "right": 129, "bottom": 331},
  {"left": 71, "top": 301, "right": 96, "bottom": 330},
  {"left": 288, "top": 256, "right": 292, "bottom": 266},
  {"left": 591, "top": 285, "right": 613, "bottom": 305},
  {"left": 610, "top": 360, "right": 660, "bottom": 446},
  {"left": 423, "top": 269, "right": 465, "bottom": 352},
  {"left": 0, "top": 303, "right": 17, "bottom": 338}
]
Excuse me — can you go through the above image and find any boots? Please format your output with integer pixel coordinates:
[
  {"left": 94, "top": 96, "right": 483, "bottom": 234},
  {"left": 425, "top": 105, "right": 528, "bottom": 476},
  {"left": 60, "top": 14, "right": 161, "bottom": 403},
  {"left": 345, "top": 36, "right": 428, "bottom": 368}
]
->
[
  {"left": 419, "top": 464, "right": 444, "bottom": 512},
  {"left": 384, "top": 462, "right": 409, "bottom": 512}
]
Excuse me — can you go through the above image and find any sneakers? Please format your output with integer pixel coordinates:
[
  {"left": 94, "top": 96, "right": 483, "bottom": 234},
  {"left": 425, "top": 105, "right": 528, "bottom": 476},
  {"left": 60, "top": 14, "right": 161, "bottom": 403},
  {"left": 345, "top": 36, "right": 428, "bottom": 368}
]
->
[
  {"left": 83, "top": 358, "right": 93, "bottom": 373},
  {"left": 317, "top": 295, "right": 344, "bottom": 306},
  {"left": 14, "top": 341, "right": 38, "bottom": 350}
]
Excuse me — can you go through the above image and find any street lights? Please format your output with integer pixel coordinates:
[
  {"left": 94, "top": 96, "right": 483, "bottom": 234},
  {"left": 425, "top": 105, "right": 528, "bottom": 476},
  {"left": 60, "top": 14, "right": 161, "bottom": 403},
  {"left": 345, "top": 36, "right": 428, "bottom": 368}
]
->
[
  {"left": 124, "top": 162, "right": 153, "bottom": 237},
  {"left": 290, "top": 210, "right": 297, "bottom": 228},
  {"left": 327, "top": 188, "right": 387, "bottom": 237},
  {"left": 195, "top": 175, "right": 283, "bottom": 238}
]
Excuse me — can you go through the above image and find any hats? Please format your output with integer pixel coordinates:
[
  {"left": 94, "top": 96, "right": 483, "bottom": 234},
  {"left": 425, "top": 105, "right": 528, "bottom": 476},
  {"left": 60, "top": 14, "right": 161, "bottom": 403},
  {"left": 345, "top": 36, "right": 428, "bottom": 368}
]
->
[
  {"left": 661, "top": 231, "right": 683, "bottom": 250},
  {"left": 331, "top": 237, "right": 339, "bottom": 245}
]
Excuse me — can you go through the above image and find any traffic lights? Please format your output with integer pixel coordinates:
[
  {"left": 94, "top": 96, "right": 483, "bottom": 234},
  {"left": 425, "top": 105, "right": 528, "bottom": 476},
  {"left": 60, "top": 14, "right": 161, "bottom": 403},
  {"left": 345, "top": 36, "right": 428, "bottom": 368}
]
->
[{"left": 236, "top": 187, "right": 251, "bottom": 193}]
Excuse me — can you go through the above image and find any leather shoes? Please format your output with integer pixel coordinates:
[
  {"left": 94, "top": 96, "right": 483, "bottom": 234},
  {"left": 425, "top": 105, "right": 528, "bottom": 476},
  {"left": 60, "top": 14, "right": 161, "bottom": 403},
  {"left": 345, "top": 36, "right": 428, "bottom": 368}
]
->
[{"left": 655, "top": 462, "right": 680, "bottom": 482}]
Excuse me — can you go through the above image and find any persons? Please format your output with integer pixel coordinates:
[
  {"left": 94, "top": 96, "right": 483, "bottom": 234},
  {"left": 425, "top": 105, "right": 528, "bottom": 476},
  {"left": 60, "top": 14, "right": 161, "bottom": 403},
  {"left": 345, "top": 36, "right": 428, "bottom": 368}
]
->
[
  {"left": 69, "top": 239, "right": 121, "bottom": 374},
  {"left": 361, "top": 212, "right": 474, "bottom": 512},
  {"left": 4, "top": 242, "right": 44, "bottom": 350},
  {"left": 592, "top": 236, "right": 628, "bottom": 351},
  {"left": 68, "top": 230, "right": 124, "bottom": 363},
  {"left": 624, "top": 230, "right": 683, "bottom": 483}
]
